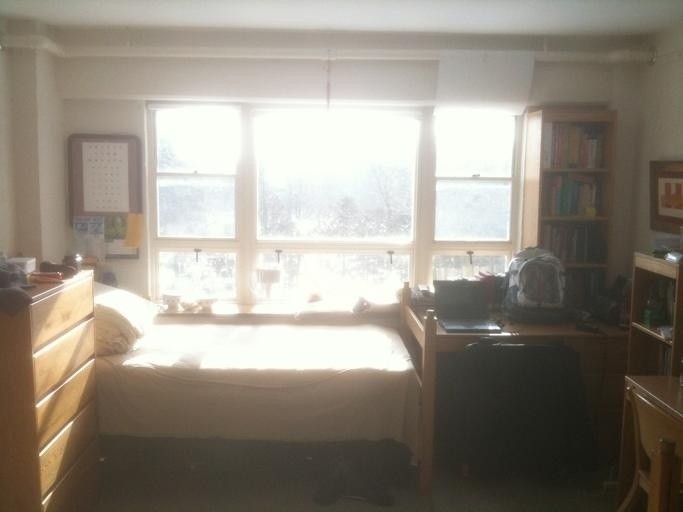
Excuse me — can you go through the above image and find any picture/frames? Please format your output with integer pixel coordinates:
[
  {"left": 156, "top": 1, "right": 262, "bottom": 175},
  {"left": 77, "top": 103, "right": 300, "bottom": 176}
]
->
[
  {"left": 649, "top": 159, "right": 683, "bottom": 232},
  {"left": 67, "top": 134, "right": 142, "bottom": 232}
]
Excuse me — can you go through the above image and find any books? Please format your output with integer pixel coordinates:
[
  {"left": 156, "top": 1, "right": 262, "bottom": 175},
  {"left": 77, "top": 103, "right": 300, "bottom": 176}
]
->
[
  {"left": 542, "top": 120, "right": 608, "bottom": 308},
  {"left": 652, "top": 234, "right": 683, "bottom": 377},
  {"left": 411, "top": 268, "right": 508, "bottom": 316}
]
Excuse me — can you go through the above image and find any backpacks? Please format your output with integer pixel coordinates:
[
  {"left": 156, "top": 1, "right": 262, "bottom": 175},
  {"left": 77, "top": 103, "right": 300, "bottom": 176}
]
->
[{"left": 496, "top": 246, "right": 569, "bottom": 324}]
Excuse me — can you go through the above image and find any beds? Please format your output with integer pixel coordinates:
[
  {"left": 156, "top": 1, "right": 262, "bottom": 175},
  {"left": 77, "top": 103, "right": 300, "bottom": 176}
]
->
[{"left": 43, "top": 258, "right": 438, "bottom": 498}]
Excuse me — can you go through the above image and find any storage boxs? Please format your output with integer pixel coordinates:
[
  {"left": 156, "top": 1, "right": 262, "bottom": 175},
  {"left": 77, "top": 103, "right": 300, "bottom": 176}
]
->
[{"left": 5, "top": 257, "right": 36, "bottom": 274}]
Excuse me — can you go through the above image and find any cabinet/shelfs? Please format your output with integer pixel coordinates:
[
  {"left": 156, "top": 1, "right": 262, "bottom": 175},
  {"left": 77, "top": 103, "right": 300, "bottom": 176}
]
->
[
  {"left": 618, "top": 252, "right": 682, "bottom": 512},
  {"left": 520, "top": 107, "right": 618, "bottom": 286}
]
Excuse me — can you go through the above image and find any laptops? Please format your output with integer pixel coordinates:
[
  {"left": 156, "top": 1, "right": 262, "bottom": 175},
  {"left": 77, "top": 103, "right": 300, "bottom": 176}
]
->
[{"left": 434, "top": 280, "right": 501, "bottom": 332}]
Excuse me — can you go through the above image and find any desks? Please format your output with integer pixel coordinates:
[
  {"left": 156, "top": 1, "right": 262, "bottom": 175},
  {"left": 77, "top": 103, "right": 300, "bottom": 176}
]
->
[
  {"left": 412, "top": 300, "right": 627, "bottom": 470},
  {"left": 619, "top": 371, "right": 682, "bottom": 512}
]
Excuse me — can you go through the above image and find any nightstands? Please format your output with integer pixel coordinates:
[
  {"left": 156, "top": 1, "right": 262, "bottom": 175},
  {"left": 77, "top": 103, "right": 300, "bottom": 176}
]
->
[{"left": 0, "top": 267, "right": 100, "bottom": 511}]
